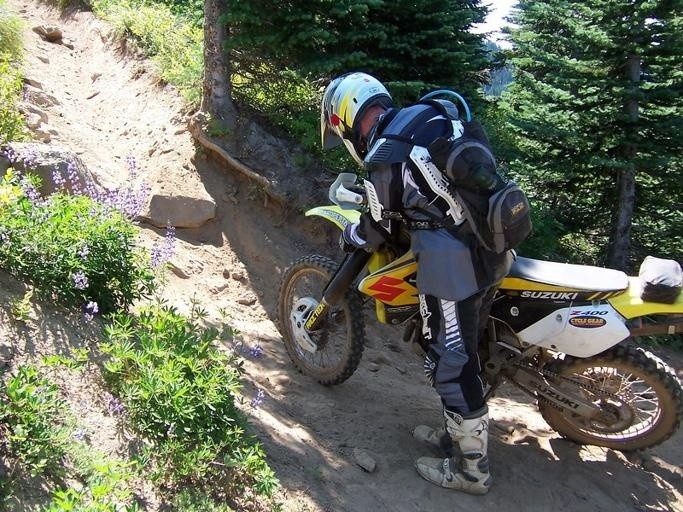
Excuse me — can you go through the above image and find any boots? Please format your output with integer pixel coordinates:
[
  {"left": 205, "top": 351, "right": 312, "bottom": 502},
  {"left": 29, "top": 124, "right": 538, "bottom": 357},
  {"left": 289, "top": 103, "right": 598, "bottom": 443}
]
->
[{"left": 409, "top": 404, "right": 494, "bottom": 497}]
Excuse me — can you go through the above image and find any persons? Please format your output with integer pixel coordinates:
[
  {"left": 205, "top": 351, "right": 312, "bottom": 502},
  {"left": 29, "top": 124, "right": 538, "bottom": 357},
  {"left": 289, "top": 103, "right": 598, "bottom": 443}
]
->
[{"left": 327, "top": 71, "right": 514, "bottom": 497}]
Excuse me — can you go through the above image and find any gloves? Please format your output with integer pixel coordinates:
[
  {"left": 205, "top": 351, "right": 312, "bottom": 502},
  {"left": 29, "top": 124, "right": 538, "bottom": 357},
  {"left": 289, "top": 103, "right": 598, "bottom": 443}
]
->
[{"left": 338, "top": 221, "right": 371, "bottom": 255}]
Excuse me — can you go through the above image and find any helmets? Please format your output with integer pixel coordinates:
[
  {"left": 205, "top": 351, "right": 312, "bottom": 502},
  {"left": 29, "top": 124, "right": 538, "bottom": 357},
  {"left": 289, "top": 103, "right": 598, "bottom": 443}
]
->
[{"left": 318, "top": 69, "right": 393, "bottom": 169}]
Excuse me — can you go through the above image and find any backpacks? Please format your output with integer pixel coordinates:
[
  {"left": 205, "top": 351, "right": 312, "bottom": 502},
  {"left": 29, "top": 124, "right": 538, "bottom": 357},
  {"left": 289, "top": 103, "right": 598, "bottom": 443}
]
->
[{"left": 376, "top": 99, "right": 533, "bottom": 256}]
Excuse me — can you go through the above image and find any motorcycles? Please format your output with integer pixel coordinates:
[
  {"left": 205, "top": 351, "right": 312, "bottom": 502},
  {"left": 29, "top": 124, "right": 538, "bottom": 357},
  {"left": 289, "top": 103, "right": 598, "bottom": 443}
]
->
[{"left": 276, "top": 172, "right": 682, "bottom": 452}]
